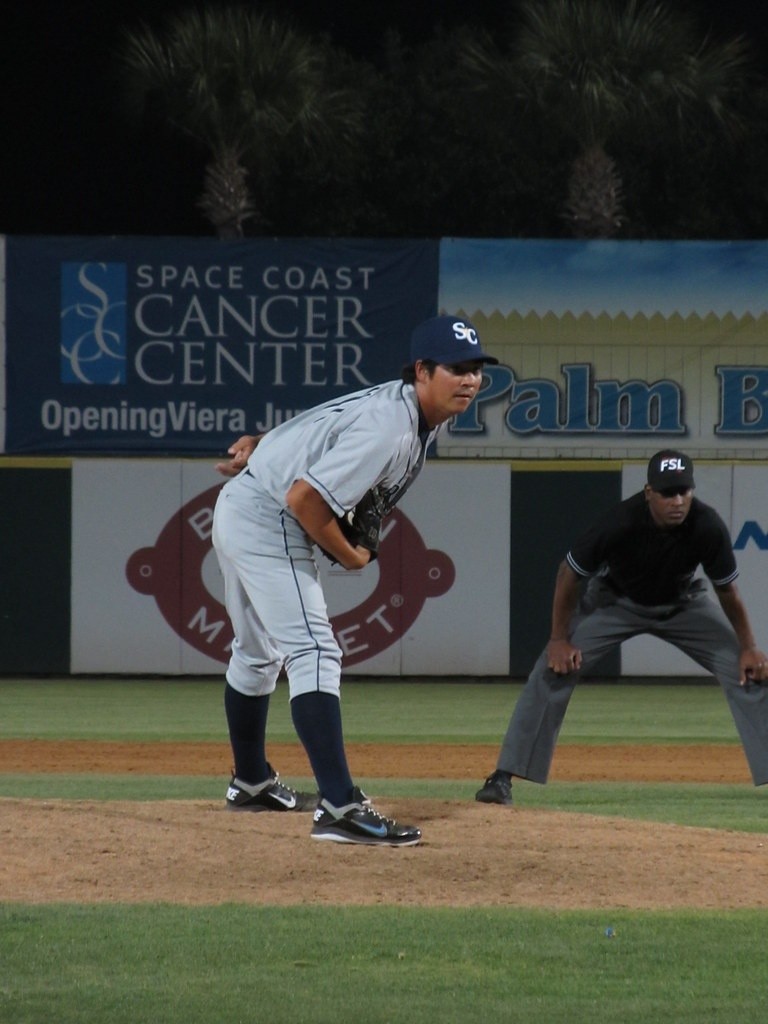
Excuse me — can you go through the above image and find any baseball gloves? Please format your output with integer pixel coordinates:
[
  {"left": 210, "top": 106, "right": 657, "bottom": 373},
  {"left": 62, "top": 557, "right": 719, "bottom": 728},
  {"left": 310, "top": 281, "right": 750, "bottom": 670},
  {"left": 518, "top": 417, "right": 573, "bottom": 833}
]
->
[{"left": 317, "top": 482, "right": 388, "bottom": 570}]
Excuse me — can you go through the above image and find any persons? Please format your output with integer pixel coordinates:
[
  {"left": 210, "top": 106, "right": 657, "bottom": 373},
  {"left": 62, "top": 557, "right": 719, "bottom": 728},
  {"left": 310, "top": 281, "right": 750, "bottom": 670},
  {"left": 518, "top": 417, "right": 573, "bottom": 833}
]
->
[
  {"left": 212, "top": 316, "right": 499, "bottom": 848},
  {"left": 476, "top": 449, "right": 768, "bottom": 805}
]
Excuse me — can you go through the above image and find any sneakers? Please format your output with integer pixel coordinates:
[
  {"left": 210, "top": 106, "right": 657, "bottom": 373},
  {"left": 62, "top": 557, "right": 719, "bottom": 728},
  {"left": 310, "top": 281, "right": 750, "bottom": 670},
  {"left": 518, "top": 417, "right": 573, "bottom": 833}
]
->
[
  {"left": 309, "top": 786, "right": 423, "bottom": 849},
  {"left": 225, "top": 762, "right": 320, "bottom": 813},
  {"left": 475, "top": 771, "right": 514, "bottom": 805}
]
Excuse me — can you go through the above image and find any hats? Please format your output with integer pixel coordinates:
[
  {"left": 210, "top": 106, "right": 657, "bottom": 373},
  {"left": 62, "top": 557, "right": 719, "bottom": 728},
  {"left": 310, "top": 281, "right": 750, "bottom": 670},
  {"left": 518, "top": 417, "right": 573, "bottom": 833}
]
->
[
  {"left": 644, "top": 449, "right": 697, "bottom": 492},
  {"left": 408, "top": 315, "right": 501, "bottom": 368}
]
